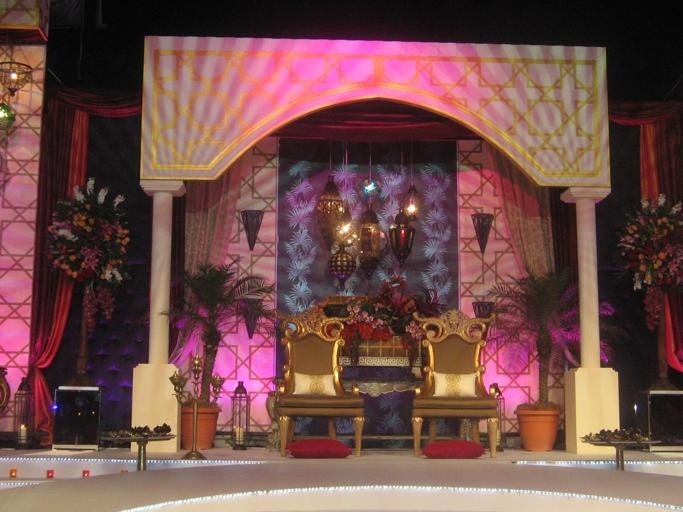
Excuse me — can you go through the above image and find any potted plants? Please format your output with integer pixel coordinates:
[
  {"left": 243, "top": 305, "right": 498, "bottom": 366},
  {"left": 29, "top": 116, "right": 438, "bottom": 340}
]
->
[
  {"left": 485, "top": 267, "right": 614, "bottom": 451},
  {"left": 162, "top": 267, "right": 276, "bottom": 450}
]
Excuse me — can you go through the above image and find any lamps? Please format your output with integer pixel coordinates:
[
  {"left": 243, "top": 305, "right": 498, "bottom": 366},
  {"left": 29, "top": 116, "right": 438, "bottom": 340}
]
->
[
  {"left": 316, "top": 138, "right": 421, "bottom": 289},
  {"left": 0, "top": 58, "right": 35, "bottom": 95},
  {"left": 230, "top": 381, "right": 249, "bottom": 451},
  {"left": 13, "top": 376, "right": 35, "bottom": 449}
]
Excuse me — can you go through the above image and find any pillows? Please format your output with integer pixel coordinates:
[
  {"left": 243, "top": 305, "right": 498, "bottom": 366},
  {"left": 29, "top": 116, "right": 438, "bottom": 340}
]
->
[
  {"left": 284, "top": 435, "right": 351, "bottom": 458},
  {"left": 422, "top": 436, "right": 483, "bottom": 458}
]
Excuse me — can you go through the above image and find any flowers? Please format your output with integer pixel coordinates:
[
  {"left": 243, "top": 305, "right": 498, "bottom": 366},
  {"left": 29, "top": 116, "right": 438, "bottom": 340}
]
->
[
  {"left": 619, "top": 189, "right": 682, "bottom": 331},
  {"left": 39, "top": 179, "right": 135, "bottom": 338}
]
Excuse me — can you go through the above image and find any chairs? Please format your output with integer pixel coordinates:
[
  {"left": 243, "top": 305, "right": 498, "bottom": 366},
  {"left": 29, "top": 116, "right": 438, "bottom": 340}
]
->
[
  {"left": 273, "top": 306, "right": 366, "bottom": 456},
  {"left": 411, "top": 309, "right": 501, "bottom": 458}
]
